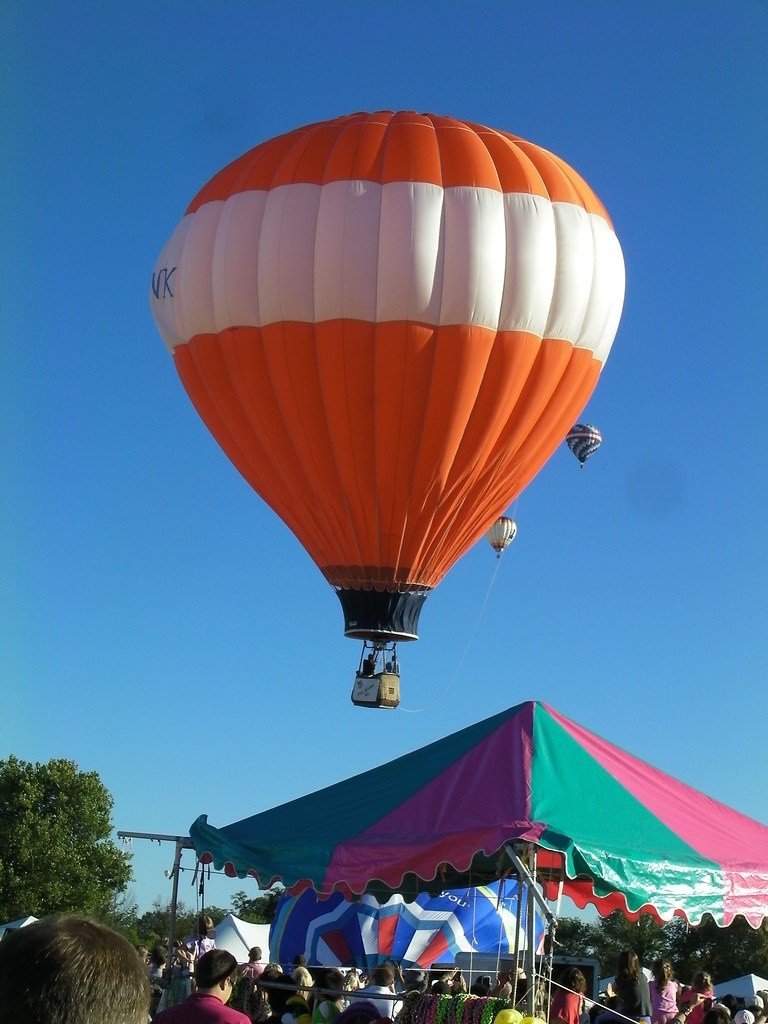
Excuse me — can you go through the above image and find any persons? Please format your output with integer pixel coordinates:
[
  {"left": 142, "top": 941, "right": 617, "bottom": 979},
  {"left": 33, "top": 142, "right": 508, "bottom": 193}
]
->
[
  {"left": 362, "top": 654, "right": 376, "bottom": 676},
  {"left": 386, "top": 655, "right": 400, "bottom": 674},
  {"left": 137, "top": 943, "right": 767, "bottom": 1024},
  {"left": 174, "top": 917, "right": 216, "bottom": 963},
  {"left": 0, "top": 917, "right": 152, "bottom": 1024}
]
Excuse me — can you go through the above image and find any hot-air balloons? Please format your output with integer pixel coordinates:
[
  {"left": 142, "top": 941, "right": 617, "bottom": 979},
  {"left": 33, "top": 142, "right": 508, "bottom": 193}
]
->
[
  {"left": 484, "top": 515, "right": 518, "bottom": 560},
  {"left": 269, "top": 876, "right": 548, "bottom": 994},
  {"left": 564, "top": 422, "right": 603, "bottom": 468},
  {"left": 149, "top": 110, "right": 629, "bottom": 712},
  {"left": 164, "top": 869, "right": 170, "bottom": 879}
]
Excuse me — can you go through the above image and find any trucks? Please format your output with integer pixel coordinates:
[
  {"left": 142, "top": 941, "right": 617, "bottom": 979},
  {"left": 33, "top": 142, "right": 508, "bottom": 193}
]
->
[{"left": 424, "top": 951, "right": 602, "bottom": 1008}]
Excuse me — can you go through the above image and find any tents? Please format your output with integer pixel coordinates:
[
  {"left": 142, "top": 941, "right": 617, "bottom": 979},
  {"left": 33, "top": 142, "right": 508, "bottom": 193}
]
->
[{"left": 166, "top": 696, "right": 768, "bottom": 1024}]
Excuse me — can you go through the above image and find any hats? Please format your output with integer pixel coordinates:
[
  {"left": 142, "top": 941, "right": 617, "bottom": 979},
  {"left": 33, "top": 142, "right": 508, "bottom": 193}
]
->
[
  {"left": 513, "top": 968, "right": 527, "bottom": 979},
  {"left": 734, "top": 1010, "right": 756, "bottom": 1024},
  {"left": 744, "top": 995, "right": 765, "bottom": 1009}
]
